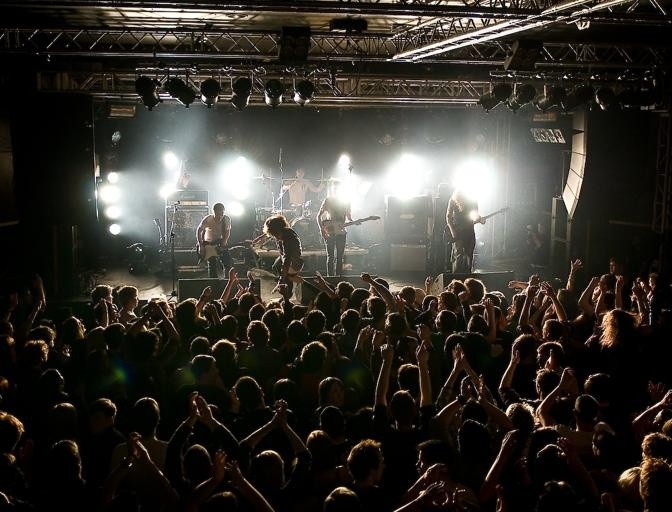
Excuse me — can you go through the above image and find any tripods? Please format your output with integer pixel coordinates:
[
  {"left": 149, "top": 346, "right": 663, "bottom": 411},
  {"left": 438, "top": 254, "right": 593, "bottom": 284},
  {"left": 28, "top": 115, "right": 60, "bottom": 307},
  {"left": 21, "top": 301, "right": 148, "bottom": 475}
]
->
[
  {"left": 258, "top": 180, "right": 284, "bottom": 249},
  {"left": 165, "top": 232, "right": 178, "bottom": 301},
  {"left": 252, "top": 192, "right": 276, "bottom": 246}
]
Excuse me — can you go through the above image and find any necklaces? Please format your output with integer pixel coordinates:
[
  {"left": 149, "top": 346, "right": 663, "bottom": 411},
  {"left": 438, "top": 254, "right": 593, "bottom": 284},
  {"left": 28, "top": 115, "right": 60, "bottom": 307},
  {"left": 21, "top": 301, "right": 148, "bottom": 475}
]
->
[{"left": 282, "top": 167, "right": 326, "bottom": 207}]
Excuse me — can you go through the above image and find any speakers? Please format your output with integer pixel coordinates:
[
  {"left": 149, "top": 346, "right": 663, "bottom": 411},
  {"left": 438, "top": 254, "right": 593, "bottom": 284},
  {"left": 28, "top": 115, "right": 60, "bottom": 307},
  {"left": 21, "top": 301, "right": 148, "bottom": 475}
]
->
[
  {"left": 431, "top": 271, "right": 515, "bottom": 297},
  {"left": 176, "top": 277, "right": 260, "bottom": 302},
  {"left": 295, "top": 275, "right": 377, "bottom": 304},
  {"left": 384, "top": 193, "right": 427, "bottom": 238}
]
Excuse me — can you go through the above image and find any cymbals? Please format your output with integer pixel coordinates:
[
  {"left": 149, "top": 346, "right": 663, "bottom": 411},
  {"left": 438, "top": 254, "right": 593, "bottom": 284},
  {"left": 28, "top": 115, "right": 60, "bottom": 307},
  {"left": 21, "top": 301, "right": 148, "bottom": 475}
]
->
[
  {"left": 316, "top": 178, "right": 339, "bottom": 184},
  {"left": 283, "top": 177, "right": 310, "bottom": 183},
  {"left": 253, "top": 176, "right": 274, "bottom": 180}
]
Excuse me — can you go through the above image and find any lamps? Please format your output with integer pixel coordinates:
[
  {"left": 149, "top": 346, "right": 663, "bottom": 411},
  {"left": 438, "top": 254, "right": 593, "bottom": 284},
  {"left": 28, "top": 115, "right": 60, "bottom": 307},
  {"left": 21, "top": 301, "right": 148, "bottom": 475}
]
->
[
  {"left": 133, "top": 68, "right": 318, "bottom": 111},
  {"left": 477, "top": 70, "right": 667, "bottom": 115}
]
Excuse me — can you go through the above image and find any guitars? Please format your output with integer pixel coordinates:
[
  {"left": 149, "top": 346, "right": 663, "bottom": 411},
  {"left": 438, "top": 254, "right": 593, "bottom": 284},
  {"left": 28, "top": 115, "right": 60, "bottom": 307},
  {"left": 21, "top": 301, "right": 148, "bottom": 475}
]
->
[
  {"left": 198, "top": 240, "right": 252, "bottom": 260},
  {"left": 443, "top": 206, "right": 509, "bottom": 244},
  {"left": 153, "top": 218, "right": 179, "bottom": 272},
  {"left": 321, "top": 215, "right": 380, "bottom": 238}
]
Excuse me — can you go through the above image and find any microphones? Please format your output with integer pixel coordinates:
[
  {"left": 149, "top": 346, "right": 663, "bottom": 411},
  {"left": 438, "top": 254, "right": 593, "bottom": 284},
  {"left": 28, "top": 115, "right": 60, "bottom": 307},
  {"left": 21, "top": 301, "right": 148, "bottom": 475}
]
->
[{"left": 170, "top": 200, "right": 181, "bottom": 207}]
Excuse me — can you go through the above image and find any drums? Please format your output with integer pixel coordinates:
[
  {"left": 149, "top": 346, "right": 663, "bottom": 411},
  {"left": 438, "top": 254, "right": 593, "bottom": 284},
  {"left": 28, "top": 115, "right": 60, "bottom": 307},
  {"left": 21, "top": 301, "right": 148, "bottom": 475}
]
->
[
  {"left": 256, "top": 208, "right": 295, "bottom": 234},
  {"left": 304, "top": 201, "right": 322, "bottom": 219}
]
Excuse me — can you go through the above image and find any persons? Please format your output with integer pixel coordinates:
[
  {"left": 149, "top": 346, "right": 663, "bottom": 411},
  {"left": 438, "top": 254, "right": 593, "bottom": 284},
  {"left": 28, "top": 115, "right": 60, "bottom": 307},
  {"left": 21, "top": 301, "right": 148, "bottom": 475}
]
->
[
  {"left": 194, "top": 203, "right": 235, "bottom": 280},
  {"left": 521, "top": 223, "right": 536, "bottom": 251},
  {"left": 1, "top": 268, "right": 671, "bottom": 512},
  {"left": 261, "top": 215, "right": 305, "bottom": 304},
  {"left": 315, "top": 181, "right": 363, "bottom": 277},
  {"left": 446, "top": 189, "right": 486, "bottom": 274},
  {"left": 533, "top": 221, "right": 549, "bottom": 248}
]
